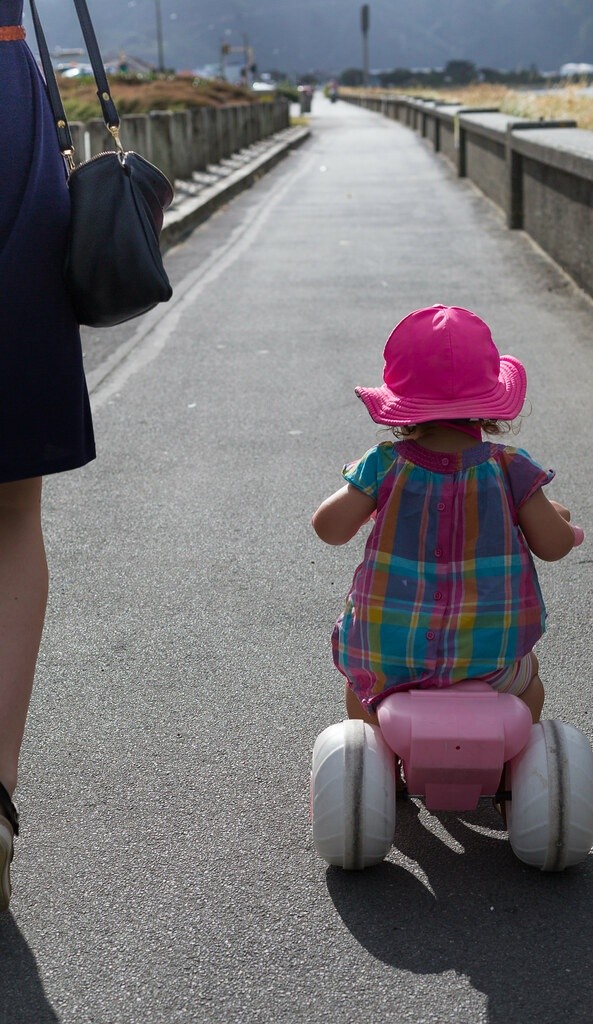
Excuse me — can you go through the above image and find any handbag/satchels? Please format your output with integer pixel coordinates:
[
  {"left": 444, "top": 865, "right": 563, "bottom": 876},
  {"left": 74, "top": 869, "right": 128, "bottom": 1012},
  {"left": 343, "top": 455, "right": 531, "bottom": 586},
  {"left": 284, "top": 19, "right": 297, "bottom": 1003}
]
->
[{"left": 63, "top": 149, "right": 176, "bottom": 329}]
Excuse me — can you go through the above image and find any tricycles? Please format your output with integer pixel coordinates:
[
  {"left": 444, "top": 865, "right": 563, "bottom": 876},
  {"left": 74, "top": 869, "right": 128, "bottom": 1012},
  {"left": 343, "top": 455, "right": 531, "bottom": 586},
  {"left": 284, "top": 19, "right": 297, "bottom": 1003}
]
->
[{"left": 308, "top": 507, "right": 593, "bottom": 874}]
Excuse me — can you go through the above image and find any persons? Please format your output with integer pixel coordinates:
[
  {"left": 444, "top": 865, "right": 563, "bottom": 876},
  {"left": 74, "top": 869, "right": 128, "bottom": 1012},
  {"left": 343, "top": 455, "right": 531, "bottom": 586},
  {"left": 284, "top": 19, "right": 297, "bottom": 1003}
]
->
[
  {"left": 1, "top": 0, "right": 97, "bottom": 907},
  {"left": 327, "top": 78, "right": 336, "bottom": 102},
  {"left": 310, "top": 303, "right": 575, "bottom": 763}
]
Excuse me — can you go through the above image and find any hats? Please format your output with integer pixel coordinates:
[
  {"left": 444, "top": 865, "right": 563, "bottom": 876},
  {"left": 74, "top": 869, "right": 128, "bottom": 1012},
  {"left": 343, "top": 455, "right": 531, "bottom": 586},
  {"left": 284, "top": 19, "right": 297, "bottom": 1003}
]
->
[{"left": 354, "top": 305, "right": 527, "bottom": 425}]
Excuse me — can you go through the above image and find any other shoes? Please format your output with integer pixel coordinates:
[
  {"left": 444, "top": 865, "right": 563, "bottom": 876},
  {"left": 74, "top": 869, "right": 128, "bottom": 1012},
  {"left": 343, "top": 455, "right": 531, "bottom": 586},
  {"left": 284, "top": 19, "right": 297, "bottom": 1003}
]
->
[
  {"left": 396, "top": 783, "right": 410, "bottom": 808},
  {"left": 492, "top": 798, "right": 503, "bottom": 817},
  {"left": 0, "top": 782, "right": 20, "bottom": 913}
]
